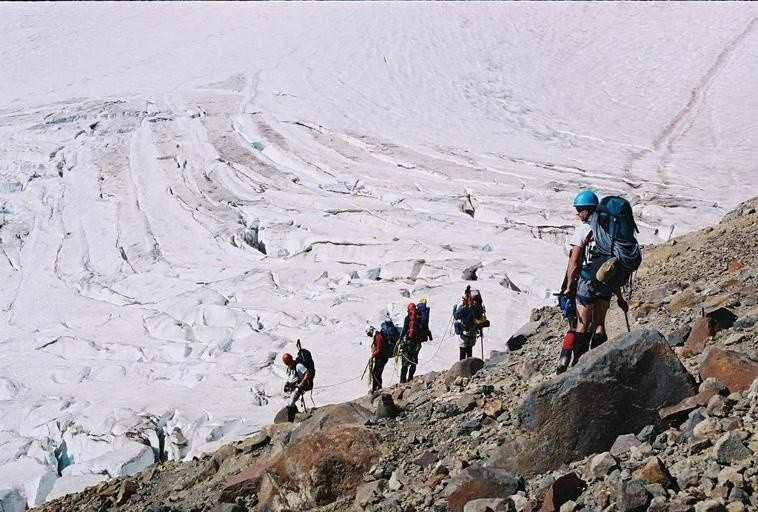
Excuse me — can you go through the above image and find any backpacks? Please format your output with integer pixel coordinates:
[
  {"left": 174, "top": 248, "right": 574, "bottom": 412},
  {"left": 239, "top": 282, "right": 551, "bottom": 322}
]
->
[
  {"left": 295, "top": 349, "right": 315, "bottom": 392},
  {"left": 381, "top": 320, "right": 401, "bottom": 355}
]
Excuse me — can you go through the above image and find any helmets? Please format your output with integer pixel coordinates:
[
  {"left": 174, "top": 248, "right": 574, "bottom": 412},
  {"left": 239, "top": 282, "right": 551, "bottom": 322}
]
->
[
  {"left": 364, "top": 325, "right": 376, "bottom": 335},
  {"left": 572, "top": 190, "right": 599, "bottom": 208},
  {"left": 282, "top": 353, "right": 293, "bottom": 363}
]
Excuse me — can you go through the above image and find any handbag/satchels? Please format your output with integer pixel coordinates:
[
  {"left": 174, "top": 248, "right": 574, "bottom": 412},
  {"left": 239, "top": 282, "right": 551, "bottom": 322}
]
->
[{"left": 581, "top": 196, "right": 642, "bottom": 289}]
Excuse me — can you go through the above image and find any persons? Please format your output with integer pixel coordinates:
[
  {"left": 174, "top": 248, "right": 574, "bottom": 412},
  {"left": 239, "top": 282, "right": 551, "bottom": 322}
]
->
[
  {"left": 283, "top": 353, "right": 312, "bottom": 422},
  {"left": 452, "top": 286, "right": 483, "bottom": 361},
  {"left": 399, "top": 304, "right": 422, "bottom": 383},
  {"left": 365, "top": 325, "right": 388, "bottom": 394},
  {"left": 564, "top": 192, "right": 628, "bottom": 367},
  {"left": 556, "top": 249, "right": 628, "bottom": 375}
]
